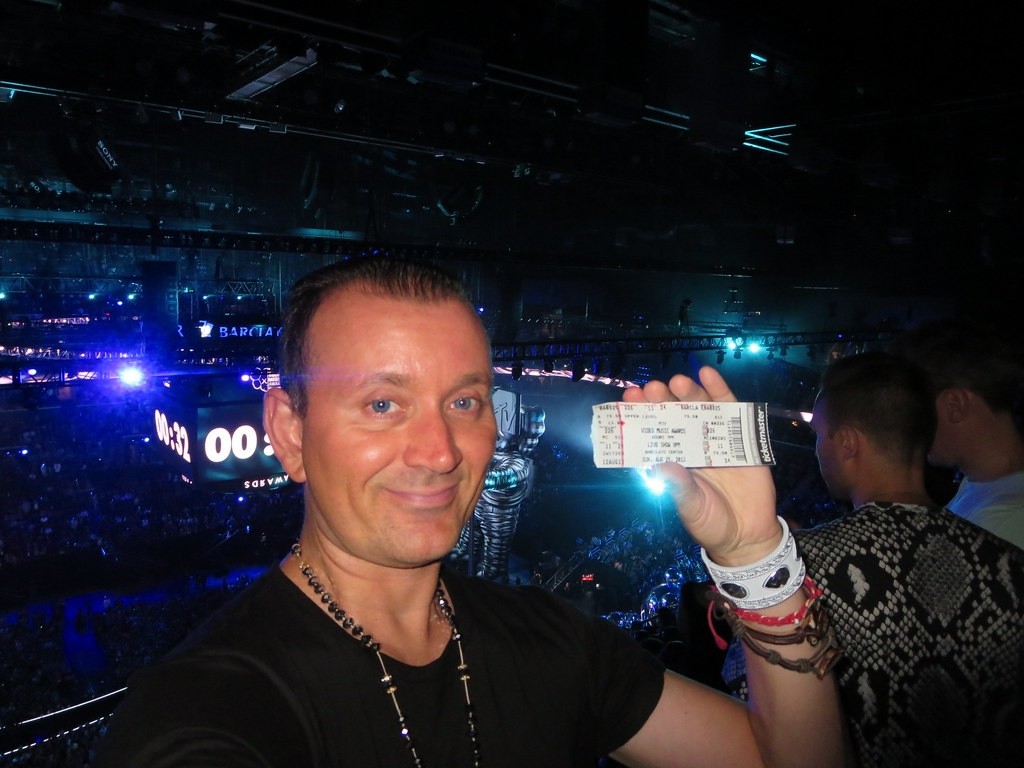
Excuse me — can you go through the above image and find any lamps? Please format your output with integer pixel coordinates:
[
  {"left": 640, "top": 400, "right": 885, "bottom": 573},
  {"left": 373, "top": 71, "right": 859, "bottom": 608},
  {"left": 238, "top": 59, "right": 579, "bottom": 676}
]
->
[
  {"left": 649, "top": 361, "right": 660, "bottom": 375},
  {"left": 806, "top": 344, "right": 817, "bottom": 357},
  {"left": 660, "top": 360, "right": 668, "bottom": 369},
  {"left": 544, "top": 359, "right": 557, "bottom": 373},
  {"left": 609, "top": 353, "right": 628, "bottom": 379},
  {"left": 779, "top": 345, "right": 789, "bottom": 356},
  {"left": 765, "top": 347, "right": 778, "bottom": 361},
  {"left": 856, "top": 341, "right": 865, "bottom": 350},
  {"left": 716, "top": 349, "right": 726, "bottom": 364},
  {"left": 510, "top": 360, "right": 525, "bottom": 381},
  {"left": 734, "top": 349, "right": 743, "bottom": 359},
  {"left": 679, "top": 352, "right": 690, "bottom": 362},
  {"left": 593, "top": 356, "right": 611, "bottom": 378},
  {"left": 571, "top": 354, "right": 593, "bottom": 383}
]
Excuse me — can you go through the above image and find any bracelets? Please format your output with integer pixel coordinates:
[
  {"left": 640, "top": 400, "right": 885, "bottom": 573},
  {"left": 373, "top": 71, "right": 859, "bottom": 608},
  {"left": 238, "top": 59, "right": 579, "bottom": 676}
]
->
[{"left": 698, "top": 515, "right": 842, "bottom": 682}]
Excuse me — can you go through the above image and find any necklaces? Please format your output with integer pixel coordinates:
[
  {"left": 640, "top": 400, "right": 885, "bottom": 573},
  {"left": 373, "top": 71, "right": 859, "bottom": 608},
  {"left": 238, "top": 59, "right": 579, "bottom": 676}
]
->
[{"left": 288, "top": 536, "right": 479, "bottom": 768}]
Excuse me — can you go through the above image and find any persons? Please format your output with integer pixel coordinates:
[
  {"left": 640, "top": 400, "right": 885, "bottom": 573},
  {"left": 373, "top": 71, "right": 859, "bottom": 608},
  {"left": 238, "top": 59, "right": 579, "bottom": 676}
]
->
[
  {"left": 0, "top": 368, "right": 302, "bottom": 768},
  {"left": 923, "top": 323, "right": 1024, "bottom": 549},
  {"left": 716, "top": 345, "right": 1024, "bottom": 768},
  {"left": 101, "top": 250, "right": 847, "bottom": 768}
]
